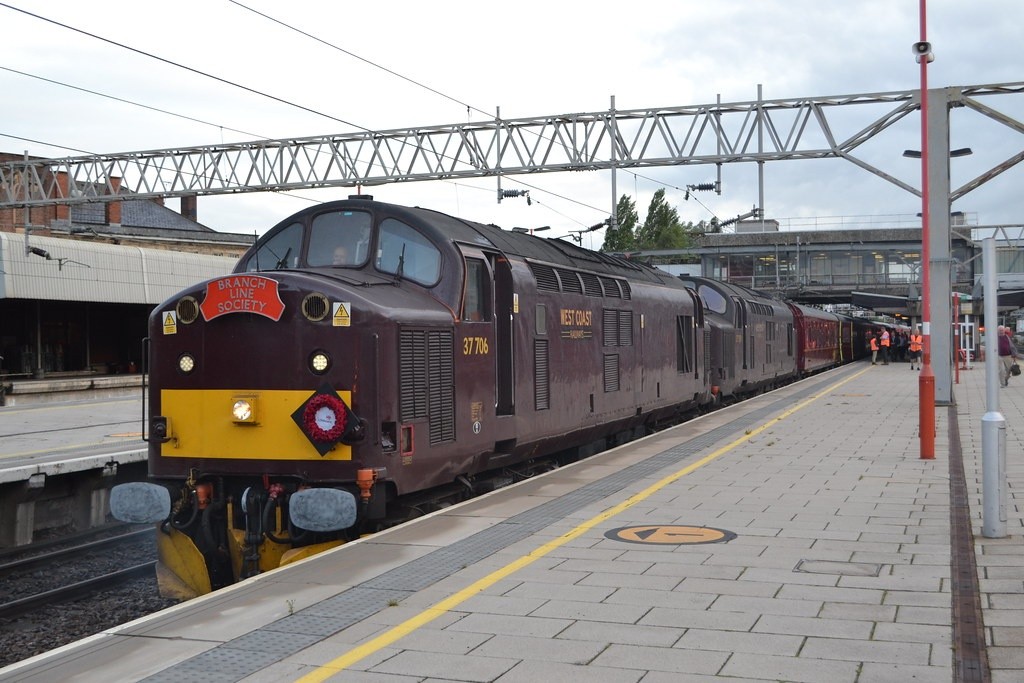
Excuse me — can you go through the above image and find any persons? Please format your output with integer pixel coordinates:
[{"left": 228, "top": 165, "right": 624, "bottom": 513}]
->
[
  {"left": 332, "top": 247, "right": 347, "bottom": 265},
  {"left": 890, "top": 329, "right": 909, "bottom": 361},
  {"left": 869, "top": 333, "right": 880, "bottom": 365},
  {"left": 979, "top": 330, "right": 985, "bottom": 362},
  {"left": 907, "top": 329, "right": 923, "bottom": 370},
  {"left": 879, "top": 325, "right": 891, "bottom": 365},
  {"left": 997, "top": 324, "right": 1018, "bottom": 389}
]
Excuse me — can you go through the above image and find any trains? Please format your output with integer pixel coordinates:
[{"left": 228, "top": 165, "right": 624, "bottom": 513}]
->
[{"left": 107, "top": 194, "right": 911, "bottom": 600}]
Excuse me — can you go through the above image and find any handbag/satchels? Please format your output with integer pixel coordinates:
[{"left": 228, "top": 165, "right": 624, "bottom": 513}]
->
[
  {"left": 1010, "top": 361, "right": 1021, "bottom": 376},
  {"left": 1010, "top": 345, "right": 1017, "bottom": 354}
]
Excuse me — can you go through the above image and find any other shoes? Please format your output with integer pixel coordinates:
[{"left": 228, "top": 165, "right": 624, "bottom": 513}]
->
[
  {"left": 872, "top": 362, "right": 878, "bottom": 365},
  {"left": 881, "top": 362, "right": 889, "bottom": 365},
  {"left": 911, "top": 365, "right": 914, "bottom": 370},
  {"left": 917, "top": 367, "right": 920, "bottom": 370}
]
